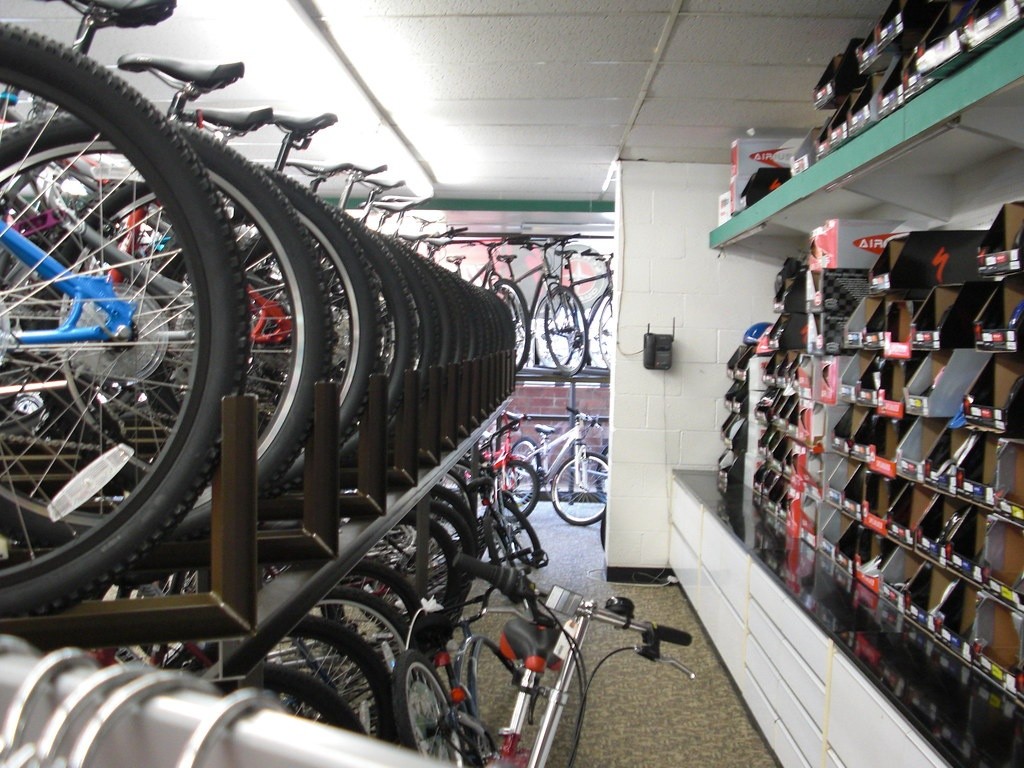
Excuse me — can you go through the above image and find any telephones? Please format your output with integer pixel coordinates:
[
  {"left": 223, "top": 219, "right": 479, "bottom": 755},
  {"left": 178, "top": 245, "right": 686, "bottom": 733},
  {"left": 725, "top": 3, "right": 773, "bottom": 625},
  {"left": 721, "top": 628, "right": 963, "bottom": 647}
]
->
[{"left": 643, "top": 333, "right": 672, "bottom": 370}]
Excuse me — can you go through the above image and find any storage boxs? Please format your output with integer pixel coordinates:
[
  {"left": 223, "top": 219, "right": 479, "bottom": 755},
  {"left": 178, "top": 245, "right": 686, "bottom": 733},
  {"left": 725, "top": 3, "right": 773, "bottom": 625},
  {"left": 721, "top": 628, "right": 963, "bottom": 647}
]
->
[{"left": 716, "top": 0, "right": 1024, "bottom": 614}]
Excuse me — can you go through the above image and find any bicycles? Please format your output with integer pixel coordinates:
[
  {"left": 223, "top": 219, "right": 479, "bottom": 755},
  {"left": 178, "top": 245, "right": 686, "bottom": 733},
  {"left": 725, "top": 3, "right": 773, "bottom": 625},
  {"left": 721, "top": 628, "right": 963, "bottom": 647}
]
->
[{"left": 0, "top": 0, "right": 704, "bottom": 768}]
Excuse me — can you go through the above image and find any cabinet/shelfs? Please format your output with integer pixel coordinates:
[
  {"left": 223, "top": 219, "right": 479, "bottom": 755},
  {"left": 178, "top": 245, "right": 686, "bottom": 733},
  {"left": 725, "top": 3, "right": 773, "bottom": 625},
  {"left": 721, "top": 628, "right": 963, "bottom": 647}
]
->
[{"left": 716, "top": 0, "right": 1024, "bottom": 768}]
